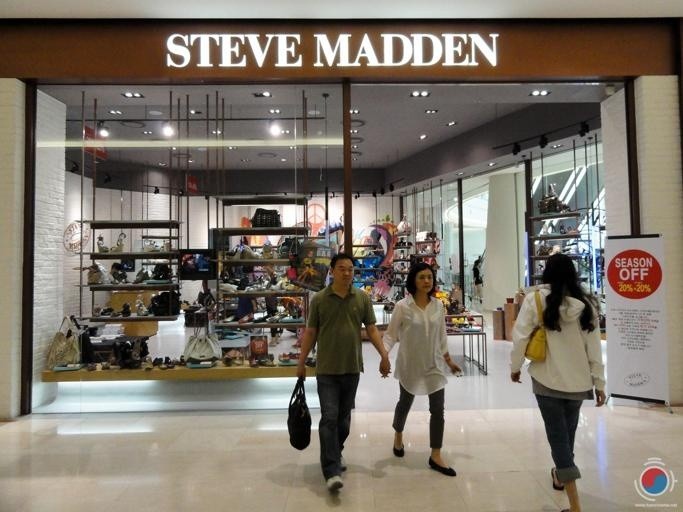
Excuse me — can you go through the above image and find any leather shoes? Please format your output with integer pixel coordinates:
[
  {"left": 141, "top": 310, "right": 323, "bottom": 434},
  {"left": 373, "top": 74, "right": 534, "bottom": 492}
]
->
[
  {"left": 394, "top": 438, "right": 404, "bottom": 458},
  {"left": 428, "top": 455, "right": 456, "bottom": 476}
]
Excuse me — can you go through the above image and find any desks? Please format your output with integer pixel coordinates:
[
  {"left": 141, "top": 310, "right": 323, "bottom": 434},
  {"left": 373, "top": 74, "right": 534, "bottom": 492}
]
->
[{"left": 383, "top": 306, "right": 487, "bottom": 375}]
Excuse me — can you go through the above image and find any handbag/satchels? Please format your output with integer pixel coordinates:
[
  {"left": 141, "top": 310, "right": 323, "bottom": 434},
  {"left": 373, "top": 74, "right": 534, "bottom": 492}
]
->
[
  {"left": 250, "top": 208, "right": 282, "bottom": 227},
  {"left": 151, "top": 290, "right": 181, "bottom": 317},
  {"left": 291, "top": 261, "right": 329, "bottom": 291},
  {"left": 153, "top": 263, "right": 172, "bottom": 281},
  {"left": 538, "top": 184, "right": 562, "bottom": 214},
  {"left": 184, "top": 333, "right": 223, "bottom": 364},
  {"left": 537, "top": 246, "right": 553, "bottom": 256},
  {"left": 45, "top": 314, "right": 82, "bottom": 371},
  {"left": 282, "top": 237, "right": 301, "bottom": 256},
  {"left": 524, "top": 289, "right": 548, "bottom": 363},
  {"left": 87, "top": 262, "right": 109, "bottom": 284},
  {"left": 287, "top": 374, "right": 311, "bottom": 450}
]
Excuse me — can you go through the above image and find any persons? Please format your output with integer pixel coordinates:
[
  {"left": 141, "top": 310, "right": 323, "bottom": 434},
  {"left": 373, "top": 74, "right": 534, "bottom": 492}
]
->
[
  {"left": 468, "top": 259, "right": 484, "bottom": 303},
  {"left": 509, "top": 253, "right": 607, "bottom": 511},
  {"left": 236, "top": 278, "right": 254, "bottom": 324},
  {"left": 379, "top": 262, "right": 465, "bottom": 476},
  {"left": 262, "top": 263, "right": 286, "bottom": 346},
  {"left": 193, "top": 280, "right": 216, "bottom": 336},
  {"left": 339, "top": 244, "right": 344, "bottom": 253},
  {"left": 297, "top": 253, "right": 391, "bottom": 492}
]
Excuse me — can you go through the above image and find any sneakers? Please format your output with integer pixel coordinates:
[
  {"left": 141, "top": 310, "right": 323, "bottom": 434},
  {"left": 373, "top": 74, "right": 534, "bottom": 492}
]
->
[
  {"left": 268, "top": 339, "right": 280, "bottom": 347},
  {"left": 327, "top": 473, "right": 342, "bottom": 492},
  {"left": 468, "top": 295, "right": 472, "bottom": 303},
  {"left": 338, "top": 453, "right": 347, "bottom": 472}
]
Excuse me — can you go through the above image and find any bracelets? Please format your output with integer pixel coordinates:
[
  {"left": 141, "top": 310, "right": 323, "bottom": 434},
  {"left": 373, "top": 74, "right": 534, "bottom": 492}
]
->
[{"left": 447, "top": 356, "right": 450, "bottom": 360}]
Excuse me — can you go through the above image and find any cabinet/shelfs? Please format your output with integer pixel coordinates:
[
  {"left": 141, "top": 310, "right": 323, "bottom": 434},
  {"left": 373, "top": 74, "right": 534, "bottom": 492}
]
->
[
  {"left": 529, "top": 214, "right": 588, "bottom": 283},
  {"left": 352, "top": 245, "right": 378, "bottom": 284},
  {"left": 415, "top": 240, "right": 445, "bottom": 286},
  {"left": 392, "top": 231, "right": 411, "bottom": 287},
  {"left": 214, "top": 227, "right": 308, "bottom": 327},
  {"left": 89, "top": 221, "right": 180, "bottom": 321}
]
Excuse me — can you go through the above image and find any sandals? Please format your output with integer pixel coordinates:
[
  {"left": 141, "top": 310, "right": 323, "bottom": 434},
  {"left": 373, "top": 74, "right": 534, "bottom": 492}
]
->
[
  {"left": 551, "top": 466, "right": 564, "bottom": 491},
  {"left": 216, "top": 269, "right": 304, "bottom": 290},
  {"left": 536, "top": 222, "right": 582, "bottom": 235},
  {"left": 86, "top": 356, "right": 188, "bottom": 371},
  {"left": 393, "top": 236, "right": 413, "bottom": 301},
  {"left": 95, "top": 233, "right": 171, "bottom": 254},
  {"left": 108, "top": 268, "right": 152, "bottom": 284},
  {"left": 223, "top": 349, "right": 317, "bottom": 367},
  {"left": 443, "top": 298, "right": 476, "bottom": 334},
  {"left": 93, "top": 298, "right": 149, "bottom": 317},
  {"left": 217, "top": 308, "right": 305, "bottom": 323}
]
